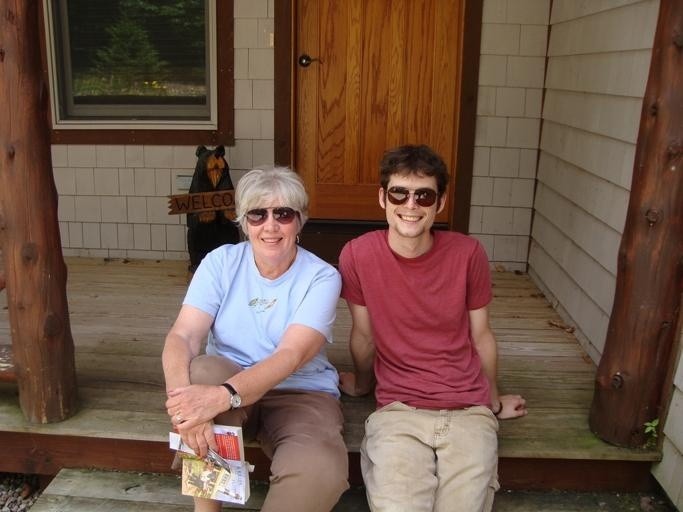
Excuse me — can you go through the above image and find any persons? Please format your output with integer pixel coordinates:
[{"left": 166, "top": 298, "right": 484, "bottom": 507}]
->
[
  {"left": 332, "top": 143, "right": 532, "bottom": 512},
  {"left": 159, "top": 163, "right": 353, "bottom": 512}
]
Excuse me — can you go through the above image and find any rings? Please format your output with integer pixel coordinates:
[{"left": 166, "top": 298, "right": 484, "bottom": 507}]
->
[{"left": 174, "top": 415, "right": 181, "bottom": 420}]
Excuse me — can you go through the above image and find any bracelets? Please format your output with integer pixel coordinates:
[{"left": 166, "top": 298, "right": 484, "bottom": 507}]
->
[{"left": 492, "top": 401, "right": 503, "bottom": 416}]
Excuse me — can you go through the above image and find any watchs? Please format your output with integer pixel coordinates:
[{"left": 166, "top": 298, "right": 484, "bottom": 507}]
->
[{"left": 219, "top": 383, "right": 240, "bottom": 412}]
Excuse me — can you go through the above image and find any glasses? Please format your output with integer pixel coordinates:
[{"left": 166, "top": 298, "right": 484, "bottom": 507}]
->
[
  {"left": 246, "top": 207, "right": 294, "bottom": 226},
  {"left": 388, "top": 187, "right": 435, "bottom": 206}
]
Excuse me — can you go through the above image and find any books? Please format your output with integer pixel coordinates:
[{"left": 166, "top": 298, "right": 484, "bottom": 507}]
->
[{"left": 181, "top": 424, "right": 251, "bottom": 505}]
